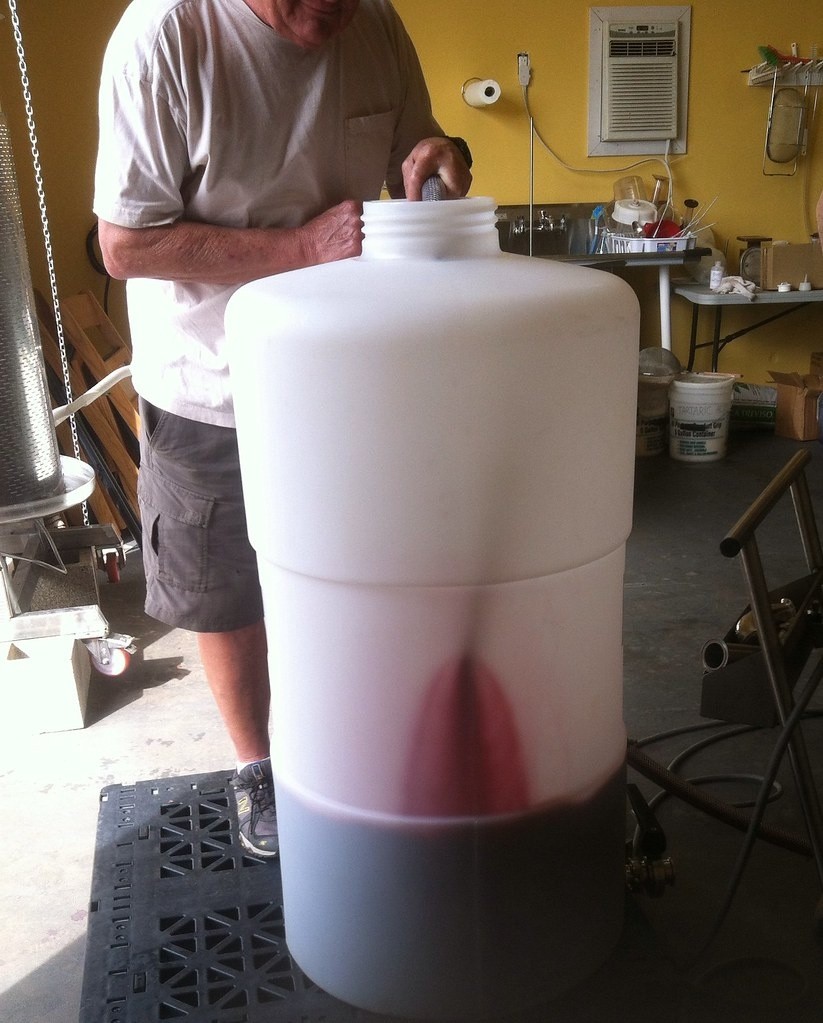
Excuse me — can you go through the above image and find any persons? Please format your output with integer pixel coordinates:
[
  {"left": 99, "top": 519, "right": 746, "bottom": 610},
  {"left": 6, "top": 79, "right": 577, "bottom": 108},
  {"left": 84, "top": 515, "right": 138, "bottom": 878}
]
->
[{"left": 89, "top": 0, "right": 473, "bottom": 862}]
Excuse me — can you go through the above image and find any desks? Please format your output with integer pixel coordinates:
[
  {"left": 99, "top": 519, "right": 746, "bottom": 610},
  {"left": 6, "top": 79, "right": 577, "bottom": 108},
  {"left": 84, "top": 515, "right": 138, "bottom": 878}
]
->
[{"left": 671, "top": 284, "right": 823, "bottom": 372}]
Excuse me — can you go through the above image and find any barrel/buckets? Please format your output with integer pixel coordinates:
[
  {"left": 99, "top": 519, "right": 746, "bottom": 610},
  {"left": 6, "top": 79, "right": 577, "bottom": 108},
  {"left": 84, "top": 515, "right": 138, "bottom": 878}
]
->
[
  {"left": 223, "top": 196, "right": 645, "bottom": 1020},
  {"left": 670, "top": 372, "right": 738, "bottom": 462},
  {"left": 634, "top": 411, "right": 669, "bottom": 456}
]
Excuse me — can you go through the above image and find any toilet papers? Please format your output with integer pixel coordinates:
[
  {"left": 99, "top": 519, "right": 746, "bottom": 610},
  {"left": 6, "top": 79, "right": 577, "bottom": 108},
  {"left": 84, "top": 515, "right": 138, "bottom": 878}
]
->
[{"left": 463, "top": 78, "right": 501, "bottom": 107}]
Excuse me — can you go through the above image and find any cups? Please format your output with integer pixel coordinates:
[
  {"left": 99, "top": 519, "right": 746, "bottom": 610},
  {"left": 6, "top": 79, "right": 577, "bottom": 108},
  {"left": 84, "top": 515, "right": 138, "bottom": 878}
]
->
[
  {"left": 777, "top": 284, "right": 791, "bottom": 292},
  {"left": 798, "top": 282, "right": 811, "bottom": 291}
]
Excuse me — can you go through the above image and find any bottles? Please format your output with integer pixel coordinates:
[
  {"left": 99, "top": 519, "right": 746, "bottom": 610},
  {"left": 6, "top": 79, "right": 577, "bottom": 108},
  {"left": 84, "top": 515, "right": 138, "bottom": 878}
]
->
[{"left": 709, "top": 261, "right": 724, "bottom": 289}]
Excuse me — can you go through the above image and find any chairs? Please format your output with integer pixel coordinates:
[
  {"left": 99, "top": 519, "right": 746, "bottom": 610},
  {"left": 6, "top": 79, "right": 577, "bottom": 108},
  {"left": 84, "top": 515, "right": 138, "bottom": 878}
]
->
[{"left": 33, "top": 288, "right": 144, "bottom": 541}]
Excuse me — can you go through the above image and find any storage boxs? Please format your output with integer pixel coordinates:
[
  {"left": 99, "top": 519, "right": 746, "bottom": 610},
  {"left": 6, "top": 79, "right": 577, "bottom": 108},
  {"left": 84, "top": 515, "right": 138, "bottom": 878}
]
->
[
  {"left": 700, "top": 572, "right": 822, "bottom": 726},
  {"left": 761, "top": 241, "right": 823, "bottom": 291},
  {"left": 766, "top": 352, "right": 823, "bottom": 440},
  {"left": 612, "top": 235, "right": 697, "bottom": 252}
]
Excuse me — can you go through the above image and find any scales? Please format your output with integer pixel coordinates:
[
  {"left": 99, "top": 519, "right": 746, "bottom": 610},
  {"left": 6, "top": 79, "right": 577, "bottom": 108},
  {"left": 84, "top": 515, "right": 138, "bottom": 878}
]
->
[{"left": 737, "top": 234, "right": 773, "bottom": 285}]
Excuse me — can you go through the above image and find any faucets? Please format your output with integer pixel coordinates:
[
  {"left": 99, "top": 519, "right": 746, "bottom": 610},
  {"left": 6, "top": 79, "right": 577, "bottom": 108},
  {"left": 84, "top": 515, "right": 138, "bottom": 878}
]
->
[{"left": 512, "top": 209, "right": 567, "bottom": 235}]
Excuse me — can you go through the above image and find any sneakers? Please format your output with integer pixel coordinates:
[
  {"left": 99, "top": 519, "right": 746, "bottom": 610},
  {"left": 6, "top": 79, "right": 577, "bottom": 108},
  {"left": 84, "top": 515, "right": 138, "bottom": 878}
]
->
[{"left": 229, "top": 758, "right": 280, "bottom": 860}]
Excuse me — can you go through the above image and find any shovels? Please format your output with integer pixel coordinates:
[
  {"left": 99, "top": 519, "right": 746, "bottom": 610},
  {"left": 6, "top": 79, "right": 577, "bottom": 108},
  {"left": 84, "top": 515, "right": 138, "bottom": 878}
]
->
[{"left": 631, "top": 220, "right": 646, "bottom": 238}]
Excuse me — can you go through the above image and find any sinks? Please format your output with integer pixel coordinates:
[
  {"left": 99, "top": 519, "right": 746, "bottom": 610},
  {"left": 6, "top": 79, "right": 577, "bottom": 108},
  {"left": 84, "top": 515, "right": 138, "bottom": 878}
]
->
[{"left": 494, "top": 217, "right": 594, "bottom": 257}]
